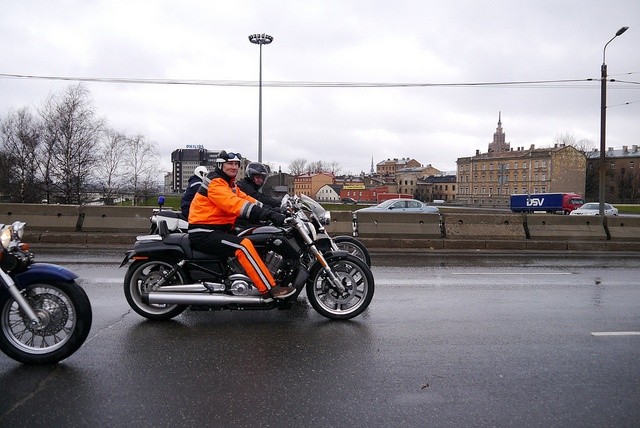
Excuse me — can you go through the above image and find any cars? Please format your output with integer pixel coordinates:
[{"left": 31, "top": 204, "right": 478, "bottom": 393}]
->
[
  {"left": 354, "top": 198, "right": 439, "bottom": 213},
  {"left": 570, "top": 202, "right": 619, "bottom": 217}
]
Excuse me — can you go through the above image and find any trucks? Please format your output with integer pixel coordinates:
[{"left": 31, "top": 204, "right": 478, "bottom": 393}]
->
[
  {"left": 377, "top": 192, "right": 414, "bottom": 204},
  {"left": 434, "top": 199, "right": 446, "bottom": 203},
  {"left": 510, "top": 193, "right": 584, "bottom": 214}
]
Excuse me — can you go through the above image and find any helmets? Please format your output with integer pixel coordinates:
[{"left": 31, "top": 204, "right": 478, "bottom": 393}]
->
[
  {"left": 246, "top": 162, "right": 272, "bottom": 187},
  {"left": 216, "top": 149, "right": 241, "bottom": 170},
  {"left": 194, "top": 165, "right": 208, "bottom": 180}
]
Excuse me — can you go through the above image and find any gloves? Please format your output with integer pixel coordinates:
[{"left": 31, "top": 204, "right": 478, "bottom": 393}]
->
[{"left": 261, "top": 203, "right": 286, "bottom": 225}]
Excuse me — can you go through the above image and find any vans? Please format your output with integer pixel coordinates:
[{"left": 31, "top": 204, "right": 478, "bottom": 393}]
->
[{"left": 341, "top": 197, "right": 359, "bottom": 205}]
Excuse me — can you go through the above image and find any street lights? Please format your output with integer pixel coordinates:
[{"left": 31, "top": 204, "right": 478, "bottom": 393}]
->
[
  {"left": 249, "top": 33, "right": 273, "bottom": 165},
  {"left": 600, "top": 26, "right": 631, "bottom": 218}
]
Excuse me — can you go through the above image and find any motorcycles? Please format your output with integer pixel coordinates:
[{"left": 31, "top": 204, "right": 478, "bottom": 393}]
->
[
  {"left": 119, "top": 194, "right": 376, "bottom": 319},
  {"left": 0, "top": 220, "right": 92, "bottom": 364},
  {"left": 144, "top": 194, "right": 372, "bottom": 288}
]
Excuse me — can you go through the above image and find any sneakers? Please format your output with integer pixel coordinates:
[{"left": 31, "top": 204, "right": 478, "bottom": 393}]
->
[{"left": 263, "top": 286, "right": 296, "bottom": 304}]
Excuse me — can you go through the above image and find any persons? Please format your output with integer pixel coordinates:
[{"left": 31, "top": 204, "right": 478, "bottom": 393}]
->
[
  {"left": 181, "top": 166, "right": 211, "bottom": 219},
  {"left": 187, "top": 150, "right": 297, "bottom": 298},
  {"left": 235, "top": 161, "right": 290, "bottom": 230}
]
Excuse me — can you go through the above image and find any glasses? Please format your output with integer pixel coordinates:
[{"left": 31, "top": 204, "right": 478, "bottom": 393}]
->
[{"left": 217, "top": 152, "right": 243, "bottom": 159}]
